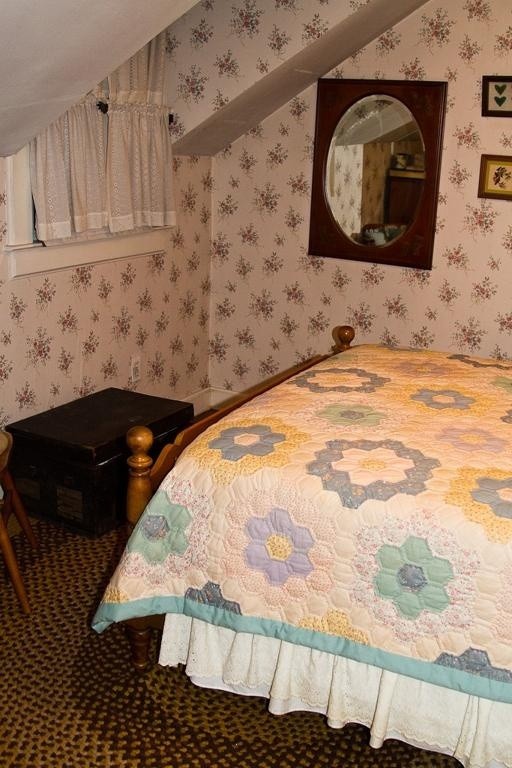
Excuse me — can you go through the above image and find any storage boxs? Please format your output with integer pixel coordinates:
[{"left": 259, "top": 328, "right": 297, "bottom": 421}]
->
[{"left": 7, "top": 388, "right": 194, "bottom": 540}]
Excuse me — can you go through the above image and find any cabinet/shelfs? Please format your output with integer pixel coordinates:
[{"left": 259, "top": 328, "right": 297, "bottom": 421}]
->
[{"left": 385, "top": 169, "right": 425, "bottom": 232}]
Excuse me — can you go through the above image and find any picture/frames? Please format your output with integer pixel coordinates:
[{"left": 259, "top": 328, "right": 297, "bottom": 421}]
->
[
  {"left": 477, "top": 153, "right": 512, "bottom": 203},
  {"left": 481, "top": 74, "right": 512, "bottom": 118}
]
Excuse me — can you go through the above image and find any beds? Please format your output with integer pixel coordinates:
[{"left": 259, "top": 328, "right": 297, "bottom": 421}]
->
[{"left": 119, "top": 325, "right": 512, "bottom": 767}]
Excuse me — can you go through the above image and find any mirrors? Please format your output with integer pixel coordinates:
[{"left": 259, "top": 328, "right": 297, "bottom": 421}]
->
[{"left": 300, "top": 77, "right": 447, "bottom": 272}]
